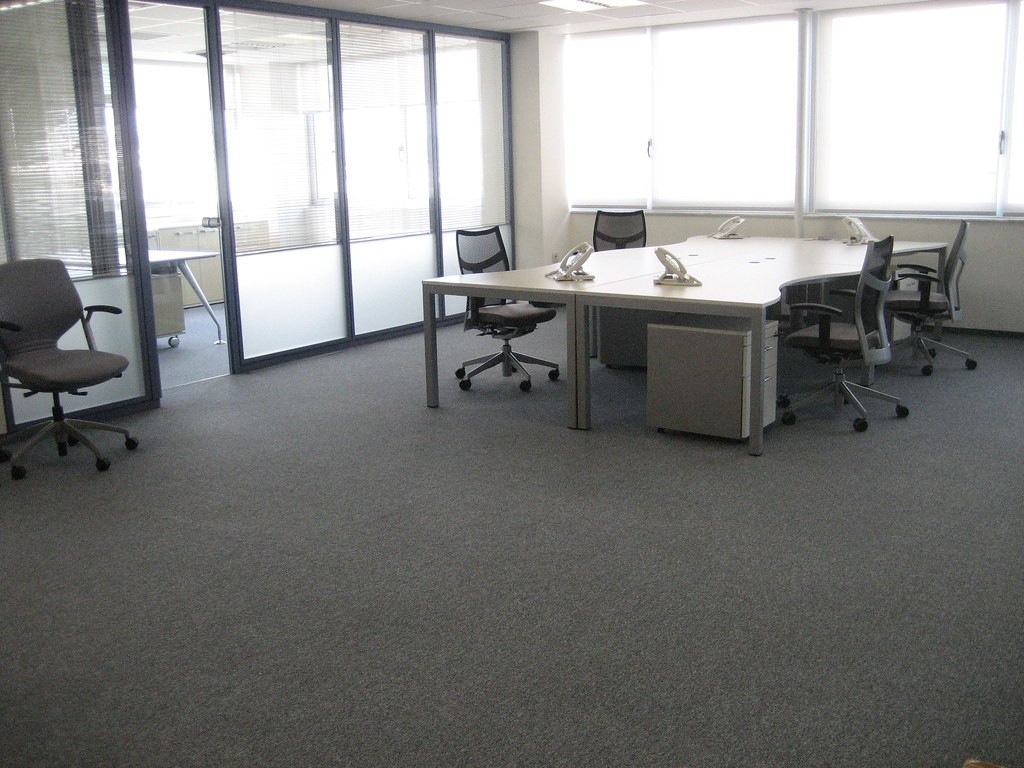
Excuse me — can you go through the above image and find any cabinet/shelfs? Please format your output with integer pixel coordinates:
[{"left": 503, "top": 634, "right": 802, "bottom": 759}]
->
[
  {"left": 151, "top": 273, "right": 186, "bottom": 347},
  {"left": 158, "top": 225, "right": 224, "bottom": 310},
  {"left": 234, "top": 220, "right": 270, "bottom": 249},
  {"left": 148, "top": 229, "right": 159, "bottom": 251},
  {"left": 646, "top": 316, "right": 778, "bottom": 444},
  {"left": 596, "top": 305, "right": 677, "bottom": 369}
]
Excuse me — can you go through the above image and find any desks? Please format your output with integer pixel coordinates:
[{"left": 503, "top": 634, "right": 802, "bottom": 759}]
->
[
  {"left": 50, "top": 248, "right": 226, "bottom": 345},
  {"left": 421, "top": 234, "right": 949, "bottom": 456}
]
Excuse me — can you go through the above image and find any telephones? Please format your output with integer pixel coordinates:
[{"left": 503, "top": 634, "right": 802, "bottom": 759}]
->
[
  {"left": 841, "top": 217, "right": 876, "bottom": 244},
  {"left": 711, "top": 215, "right": 745, "bottom": 239},
  {"left": 653, "top": 246, "right": 698, "bottom": 287},
  {"left": 552, "top": 241, "right": 596, "bottom": 282}
]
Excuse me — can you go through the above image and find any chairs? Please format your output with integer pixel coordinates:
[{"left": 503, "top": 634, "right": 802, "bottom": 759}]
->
[
  {"left": 592, "top": 209, "right": 647, "bottom": 252},
  {"left": 0, "top": 259, "right": 140, "bottom": 480},
  {"left": 777, "top": 235, "right": 909, "bottom": 432},
  {"left": 883, "top": 220, "right": 977, "bottom": 376},
  {"left": 454, "top": 226, "right": 560, "bottom": 392}
]
